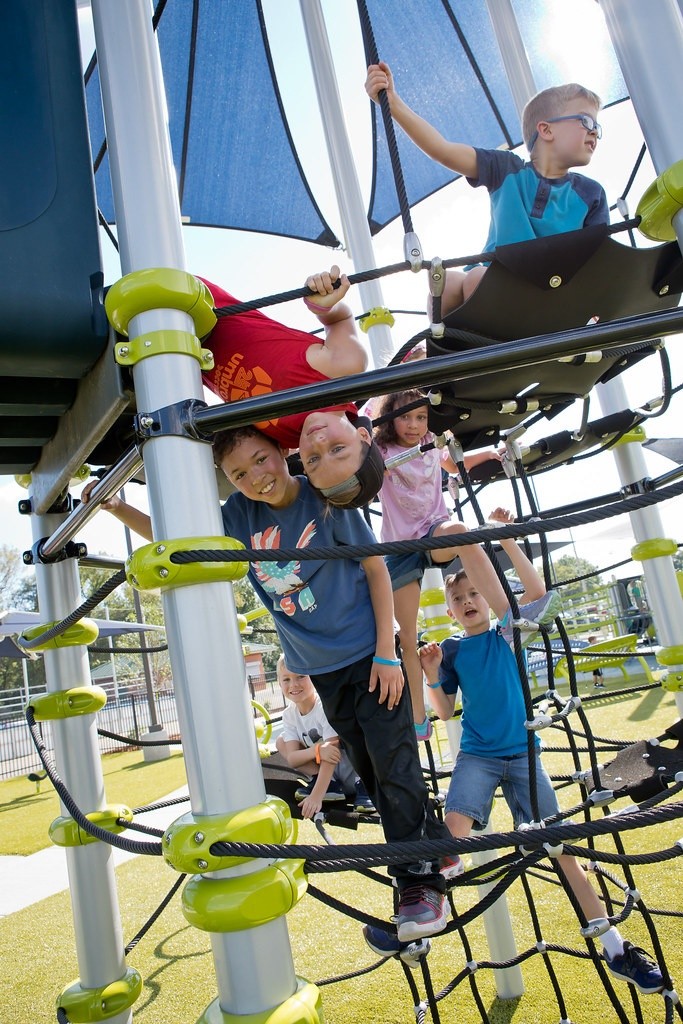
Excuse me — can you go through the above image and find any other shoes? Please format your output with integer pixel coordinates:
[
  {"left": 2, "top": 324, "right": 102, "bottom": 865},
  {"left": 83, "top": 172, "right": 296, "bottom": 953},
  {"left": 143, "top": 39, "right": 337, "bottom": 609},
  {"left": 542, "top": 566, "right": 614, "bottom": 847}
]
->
[{"left": 593, "top": 682, "right": 606, "bottom": 688}]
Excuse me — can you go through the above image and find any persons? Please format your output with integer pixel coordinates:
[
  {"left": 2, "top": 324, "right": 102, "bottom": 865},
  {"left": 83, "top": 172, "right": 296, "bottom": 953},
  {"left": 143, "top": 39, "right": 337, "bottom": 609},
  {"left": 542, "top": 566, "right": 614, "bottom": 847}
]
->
[
  {"left": 194, "top": 265, "right": 386, "bottom": 511},
  {"left": 362, "top": 508, "right": 674, "bottom": 995},
  {"left": 364, "top": 62, "right": 613, "bottom": 326},
  {"left": 588, "top": 637, "right": 607, "bottom": 689},
  {"left": 275, "top": 652, "right": 376, "bottom": 819},
  {"left": 81, "top": 424, "right": 465, "bottom": 943},
  {"left": 373, "top": 388, "right": 561, "bottom": 741}
]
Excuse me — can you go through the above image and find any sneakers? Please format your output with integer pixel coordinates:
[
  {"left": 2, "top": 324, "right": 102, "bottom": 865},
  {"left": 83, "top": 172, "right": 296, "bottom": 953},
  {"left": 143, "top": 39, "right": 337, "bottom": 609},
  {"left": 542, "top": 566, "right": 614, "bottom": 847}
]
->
[
  {"left": 354, "top": 778, "right": 378, "bottom": 812},
  {"left": 390, "top": 854, "right": 466, "bottom": 887},
  {"left": 362, "top": 924, "right": 428, "bottom": 968},
  {"left": 390, "top": 887, "right": 453, "bottom": 941},
  {"left": 295, "top": 773, "right": 346, "bottom": 801},
  {"left": 499, "top": 589, "right": 560, "bottom": 655},
  {"left": 413, "top": 717, "right": 434, "bottom": 740},
  {"left": 602, "top": 940, "right": 668, "bottom": 994}
]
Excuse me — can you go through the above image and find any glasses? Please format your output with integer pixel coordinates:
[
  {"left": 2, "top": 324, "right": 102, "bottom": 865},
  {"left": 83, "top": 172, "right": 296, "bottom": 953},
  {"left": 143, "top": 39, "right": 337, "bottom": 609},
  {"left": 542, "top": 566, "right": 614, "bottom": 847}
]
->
[{"left": 527, "top": 114, "right": 602, "bottom": 148}]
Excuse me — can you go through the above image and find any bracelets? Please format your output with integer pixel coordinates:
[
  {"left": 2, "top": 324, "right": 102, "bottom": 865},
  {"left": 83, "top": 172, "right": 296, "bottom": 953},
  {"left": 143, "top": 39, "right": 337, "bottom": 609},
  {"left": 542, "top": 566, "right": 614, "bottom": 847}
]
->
[
  {"left": 424, "top": 677, "right": 442, "bottom": 688},
  {"left": 372, "top": 655, "right": 401, "bottom": 666},
  {"left": 315, "top": 744, "right": 321, "bottom": 764},
  {"left": 303, "top": 298, "right": 333, "bottom": 311}
]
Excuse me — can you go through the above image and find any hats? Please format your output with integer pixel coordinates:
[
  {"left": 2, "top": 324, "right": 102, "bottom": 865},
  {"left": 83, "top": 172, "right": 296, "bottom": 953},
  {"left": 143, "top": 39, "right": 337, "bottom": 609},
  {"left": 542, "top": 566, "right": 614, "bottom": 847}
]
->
[{"left": 307, "top": 415, "right": 386, "bottom": 511}]
